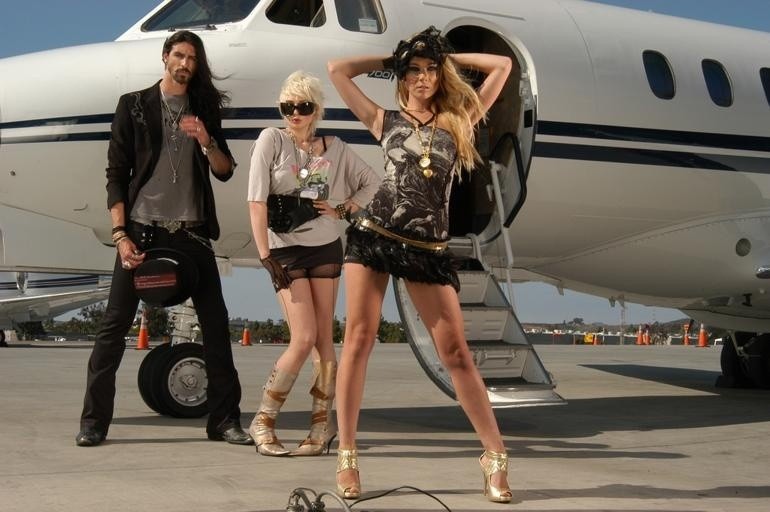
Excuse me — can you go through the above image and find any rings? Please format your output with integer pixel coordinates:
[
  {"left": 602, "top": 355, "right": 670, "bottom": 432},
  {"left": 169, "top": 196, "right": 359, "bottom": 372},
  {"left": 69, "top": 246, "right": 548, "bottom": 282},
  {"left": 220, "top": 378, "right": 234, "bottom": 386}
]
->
[
  {"left": 134, "top": 250, "right": 141, "bottom": 254},
  {"left": 131, "top": 254, "right": 138, "bottom": 260},
  {"left": 196, "top": 127, "right": 201, "bottom": 133},
  {"left": 122, "top": 261, "right": 132, "bottom": 268},
  {"left": 195, "top": 116, "right": 199, "bottom": 123}
]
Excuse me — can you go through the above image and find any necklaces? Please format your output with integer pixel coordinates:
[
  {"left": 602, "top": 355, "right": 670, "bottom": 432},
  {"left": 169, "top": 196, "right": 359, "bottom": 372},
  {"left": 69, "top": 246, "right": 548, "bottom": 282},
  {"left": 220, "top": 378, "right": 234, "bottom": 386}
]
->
[
  {"left": 160, "top": 100, "right": 193, "bottom": 184},
  {"left": 159, "top": 85, "right": 186, "bottom": 144},
  {"left": 403, "top": 103, "right": 438, "bottom": 179},
  {"left": 287, "top": 130, "right": 314, "bottom": 183}
]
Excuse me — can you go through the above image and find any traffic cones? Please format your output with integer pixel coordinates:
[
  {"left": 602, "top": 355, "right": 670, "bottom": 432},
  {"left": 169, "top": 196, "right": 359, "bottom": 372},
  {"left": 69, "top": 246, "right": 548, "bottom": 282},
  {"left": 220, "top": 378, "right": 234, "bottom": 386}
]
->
[
  {"left": 695, "top": 321, "right": 711, "bottom": 349},
  {"left": 133, "top": 308, "right": 152, "bottom": 351},
  {"left": 634, "top": 323, "right": 643, "bottom": 344},
  {"left": 644, "top": 326, "right": 651, "bottom": 346},
  {"left": 242, "top": 318, "right": 252, "bottom": 347},
  {"left": 683, "top": 327, "right": 690, "bottom": 345},
  {"left": 593, "top": 334, "right": 598, "bottom": 345}
]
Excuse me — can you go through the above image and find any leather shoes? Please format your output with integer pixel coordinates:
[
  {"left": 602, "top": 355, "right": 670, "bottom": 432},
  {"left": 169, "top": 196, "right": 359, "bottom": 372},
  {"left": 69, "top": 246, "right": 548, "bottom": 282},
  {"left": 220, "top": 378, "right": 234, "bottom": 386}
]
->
[{"left": 205, "top": 414, "right": 256, "bottom": 445}]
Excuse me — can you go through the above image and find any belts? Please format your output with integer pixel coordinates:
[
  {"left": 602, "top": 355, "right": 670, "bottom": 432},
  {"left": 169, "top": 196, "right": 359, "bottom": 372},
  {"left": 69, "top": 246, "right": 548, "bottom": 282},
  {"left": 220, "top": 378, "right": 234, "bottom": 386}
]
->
[
  {"left": 73, "top": 430, "right": 107, "bottom": 448},
  {"left": 128, "top": 214, "right": 202, "bottom": 235}
]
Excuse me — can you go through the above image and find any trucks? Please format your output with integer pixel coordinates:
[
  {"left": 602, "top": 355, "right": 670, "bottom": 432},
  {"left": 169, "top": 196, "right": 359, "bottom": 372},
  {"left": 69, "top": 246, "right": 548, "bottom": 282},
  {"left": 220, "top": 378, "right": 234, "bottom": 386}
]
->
[
  {"left": 714, "top": 337, "right": 723, "bottom": 345},
  {"left": 47, "top": 336, "right": 66, "bottom": 342}
]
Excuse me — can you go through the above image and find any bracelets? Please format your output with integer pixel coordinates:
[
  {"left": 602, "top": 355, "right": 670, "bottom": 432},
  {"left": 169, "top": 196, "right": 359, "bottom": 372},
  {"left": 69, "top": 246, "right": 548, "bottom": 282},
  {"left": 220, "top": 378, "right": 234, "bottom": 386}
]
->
[
  {"left": 201, "top": 137, "right": 218, "bottom": 155},
  {"left": 336, "top": 204, "right": 347, "bottom": 220},
  {"left": 110, "top": 226, "right": 128, "bottom": 245}
]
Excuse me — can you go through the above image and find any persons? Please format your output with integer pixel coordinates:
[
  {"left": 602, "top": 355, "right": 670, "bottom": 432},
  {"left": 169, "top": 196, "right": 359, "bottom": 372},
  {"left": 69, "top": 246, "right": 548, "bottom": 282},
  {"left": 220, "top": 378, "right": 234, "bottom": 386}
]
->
[
  {"left": 77, "top": 28, "right": 253, "bottom": 447},
  {"left": 249, "top": 71, "right": 383, "bottom": 458},
  {"left": 326, "top": 26, "right": 514, "bottom": 502}
]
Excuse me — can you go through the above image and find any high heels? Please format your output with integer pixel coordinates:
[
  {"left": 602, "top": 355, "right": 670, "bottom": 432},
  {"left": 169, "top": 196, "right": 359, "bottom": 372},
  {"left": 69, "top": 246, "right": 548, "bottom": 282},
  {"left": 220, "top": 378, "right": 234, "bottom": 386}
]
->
[
  {"left": 335, "top": 446, "right": 362, "bottom": 501},
  {"left": 478, "top": 448, "right": 514, "bottom": 505}
]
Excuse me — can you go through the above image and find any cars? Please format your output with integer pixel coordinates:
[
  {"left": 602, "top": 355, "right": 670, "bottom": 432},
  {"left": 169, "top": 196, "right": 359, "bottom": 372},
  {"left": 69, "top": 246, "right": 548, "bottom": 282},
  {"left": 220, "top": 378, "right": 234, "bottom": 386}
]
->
[{"left": 87, "top": 333, "right": 151, "bottom": 343}]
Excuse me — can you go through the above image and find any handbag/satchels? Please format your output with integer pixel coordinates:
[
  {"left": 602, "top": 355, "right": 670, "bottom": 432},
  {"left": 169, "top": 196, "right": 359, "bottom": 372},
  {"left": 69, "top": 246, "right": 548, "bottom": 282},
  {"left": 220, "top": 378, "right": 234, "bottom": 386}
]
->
[
  {"left": 131, "top": 245, "right": 218, "bottom": 310},
  {"left": 265, "top": 128, "right": 326, "bottom": 236}
]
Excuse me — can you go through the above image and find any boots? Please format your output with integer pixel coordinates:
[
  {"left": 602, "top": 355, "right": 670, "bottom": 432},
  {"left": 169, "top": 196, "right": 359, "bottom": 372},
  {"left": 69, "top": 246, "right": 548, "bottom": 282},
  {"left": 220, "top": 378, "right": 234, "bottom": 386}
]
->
[
  {"left": 285, "top": 358, "right": 337, "bottom": 458},
  {"left": 246, "top": 362, "right": 301, "bottom": 458}
]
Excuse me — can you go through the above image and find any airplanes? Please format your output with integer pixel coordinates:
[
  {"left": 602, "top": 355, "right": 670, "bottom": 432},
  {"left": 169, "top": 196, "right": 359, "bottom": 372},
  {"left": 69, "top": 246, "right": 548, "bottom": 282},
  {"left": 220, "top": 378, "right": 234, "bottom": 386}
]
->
[{"left": 1, "top": 0, "right": 770, "bottom": 420}]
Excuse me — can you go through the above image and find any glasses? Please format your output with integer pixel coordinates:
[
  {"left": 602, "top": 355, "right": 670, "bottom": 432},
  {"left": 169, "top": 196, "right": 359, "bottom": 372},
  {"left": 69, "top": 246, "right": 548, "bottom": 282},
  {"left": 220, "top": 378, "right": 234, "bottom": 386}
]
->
[
  {"left": 397, "top": 38, "right": 429, "bottom": 64},
  {"left": 279, "top": 101, "right": 317, "bottom": 117}
]
